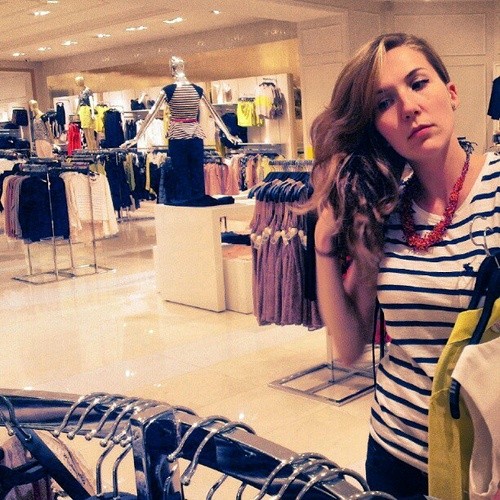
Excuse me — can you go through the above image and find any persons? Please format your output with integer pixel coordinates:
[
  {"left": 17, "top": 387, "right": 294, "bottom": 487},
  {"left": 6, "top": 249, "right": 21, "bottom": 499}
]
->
[
  {"left": 74, "top": 76, "right": 100, "bottom": 151},
  {"left": 30, "top": 99, "right": 54, "bottom": 158},
  {"left": 291, "top": 34, "right": 500, "bottom": 500},
  {"left": 125, "top": 53, "right": 244, "bottom": 201}
]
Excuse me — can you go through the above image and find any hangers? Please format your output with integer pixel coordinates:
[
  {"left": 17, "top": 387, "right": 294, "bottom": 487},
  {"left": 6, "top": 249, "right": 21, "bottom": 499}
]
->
[
  {"left": 48, "top": 389, "right": 397, "bottom": 499},
  {"left": 438, "top": 215, "right": 500, "bottom": 419},
  {"left": 14, "top": 146, "right": 314, "bottom": 205}
]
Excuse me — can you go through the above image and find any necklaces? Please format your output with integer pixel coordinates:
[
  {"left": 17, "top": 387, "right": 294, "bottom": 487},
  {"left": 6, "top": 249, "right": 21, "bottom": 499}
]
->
[{"left": 400, "top": 135, "right": 477, "bottom": 252}]
